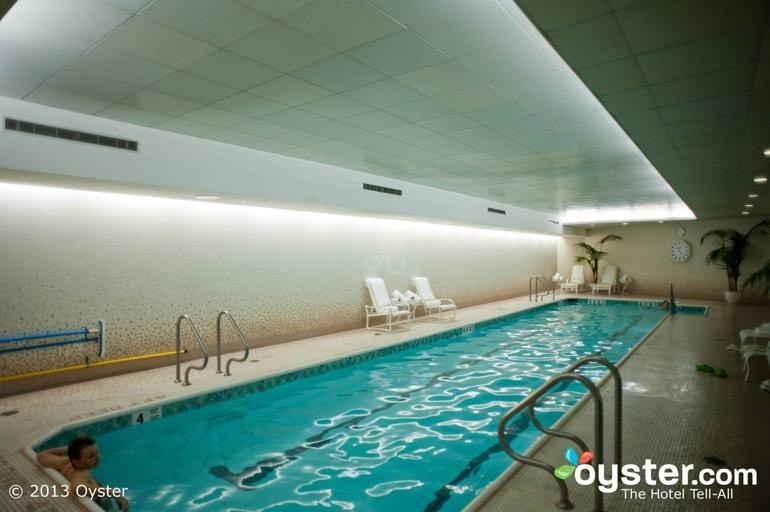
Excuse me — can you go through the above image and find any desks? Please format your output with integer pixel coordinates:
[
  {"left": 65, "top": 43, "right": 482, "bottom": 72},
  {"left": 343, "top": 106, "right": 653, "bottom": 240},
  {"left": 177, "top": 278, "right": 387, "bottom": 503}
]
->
[{"left": 619, "top": 275, "right": 634, "bottom": 296}]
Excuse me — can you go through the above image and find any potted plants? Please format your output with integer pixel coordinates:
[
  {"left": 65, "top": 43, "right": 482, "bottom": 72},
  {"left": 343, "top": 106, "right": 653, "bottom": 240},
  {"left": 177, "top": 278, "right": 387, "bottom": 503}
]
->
[{"left": 701, "top": 221, "right": 769, "bottom": 304}]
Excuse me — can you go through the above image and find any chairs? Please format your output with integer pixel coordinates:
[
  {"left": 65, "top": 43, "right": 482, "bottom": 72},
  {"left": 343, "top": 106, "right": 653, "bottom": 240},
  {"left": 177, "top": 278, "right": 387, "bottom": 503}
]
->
[
  {"left": 363, "top": 275, "right": 457, "bottom": 333},
  {"left": 562, "top": 265, "right": 620, "bottom": 297},
  {"left": 737, "top": 325, "right": 770, "bottom": 382}
]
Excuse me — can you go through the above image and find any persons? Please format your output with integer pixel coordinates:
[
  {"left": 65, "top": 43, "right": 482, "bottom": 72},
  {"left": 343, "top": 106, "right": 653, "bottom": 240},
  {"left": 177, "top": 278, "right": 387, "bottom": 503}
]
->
[
  {"left": 34, "top": 435, "right": 133, "bottom": 512},
  {"left": 659, "top": 299, "right": 672, "bottom": 313}
]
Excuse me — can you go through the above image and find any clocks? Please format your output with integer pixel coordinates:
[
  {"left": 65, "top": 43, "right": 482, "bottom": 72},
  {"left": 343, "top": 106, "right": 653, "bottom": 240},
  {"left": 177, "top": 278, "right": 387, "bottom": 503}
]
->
[{"left": 670, "top": 238, "right": 692, "bottom": 264}]
